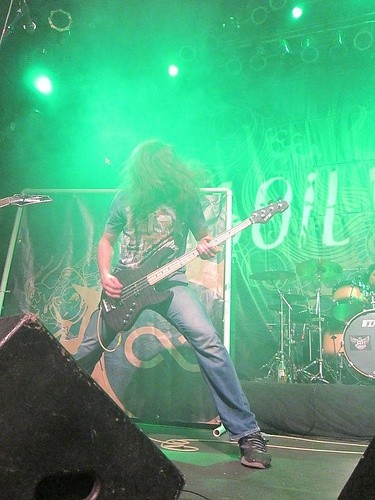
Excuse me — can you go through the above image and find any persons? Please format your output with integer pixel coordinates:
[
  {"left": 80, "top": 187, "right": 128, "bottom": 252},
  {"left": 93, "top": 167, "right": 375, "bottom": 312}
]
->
[
  {"left": 352, "top": 263, "right": 375, "bottom": 296},
  {"left": 73, "top": 141, "right": 271, "bottom": 469}
]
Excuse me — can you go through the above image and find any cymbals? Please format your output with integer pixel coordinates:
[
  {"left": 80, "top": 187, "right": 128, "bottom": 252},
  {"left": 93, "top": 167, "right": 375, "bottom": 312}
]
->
[
  {"left": 298, "top": 259, "right": 344, "bottom": 282},
  {"left": 249, "top": 270, "right": 301, "bottom": 281},
  {"left": 268, "top": 294, "right": 346, "bottom": 328}
]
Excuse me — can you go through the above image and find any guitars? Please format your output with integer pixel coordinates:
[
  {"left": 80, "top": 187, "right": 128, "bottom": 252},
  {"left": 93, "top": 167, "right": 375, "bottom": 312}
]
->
[
  {"left": 99, "top": 196, "right": 289, "bottom": 333},
  {"left": 0, "top": 193, "right": 53, "bottom": 207}
]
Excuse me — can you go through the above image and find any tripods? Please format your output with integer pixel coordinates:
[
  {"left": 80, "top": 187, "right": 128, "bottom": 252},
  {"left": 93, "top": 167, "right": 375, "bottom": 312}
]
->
[{"left": 257, "top": 269, "right": 355, "bottom": 386}]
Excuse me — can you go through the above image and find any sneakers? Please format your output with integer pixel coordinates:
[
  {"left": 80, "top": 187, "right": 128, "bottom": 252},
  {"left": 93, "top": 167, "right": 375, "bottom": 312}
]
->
[{"left": 238, "top": 433, "right": 272, "bottom": 469}]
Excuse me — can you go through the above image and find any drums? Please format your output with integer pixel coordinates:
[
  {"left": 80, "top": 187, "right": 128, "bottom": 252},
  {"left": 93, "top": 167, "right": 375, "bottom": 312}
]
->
[
  {"left": 332, "top": 281, "right": 370, "bottom": 325},
  {"left": 344, "top": 309, "right": 375, "bottom": 384},
  {"left": 323, "top": 326, "right": 344, "bottom": 356},
  {"left": 369, "top": 269, "right": 375, "bottom": 288}
]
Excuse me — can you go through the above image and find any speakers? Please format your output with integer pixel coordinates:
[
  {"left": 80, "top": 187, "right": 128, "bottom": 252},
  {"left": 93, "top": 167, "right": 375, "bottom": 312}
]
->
[{"left": 0, "top": 312, "right": 186, "bottom": 500}]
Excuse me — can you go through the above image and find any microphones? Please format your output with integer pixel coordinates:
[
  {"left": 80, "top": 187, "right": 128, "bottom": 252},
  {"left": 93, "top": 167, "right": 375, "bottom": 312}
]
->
[{"left": 19, "top": 0, "right": 36, "bottom": 32}]
